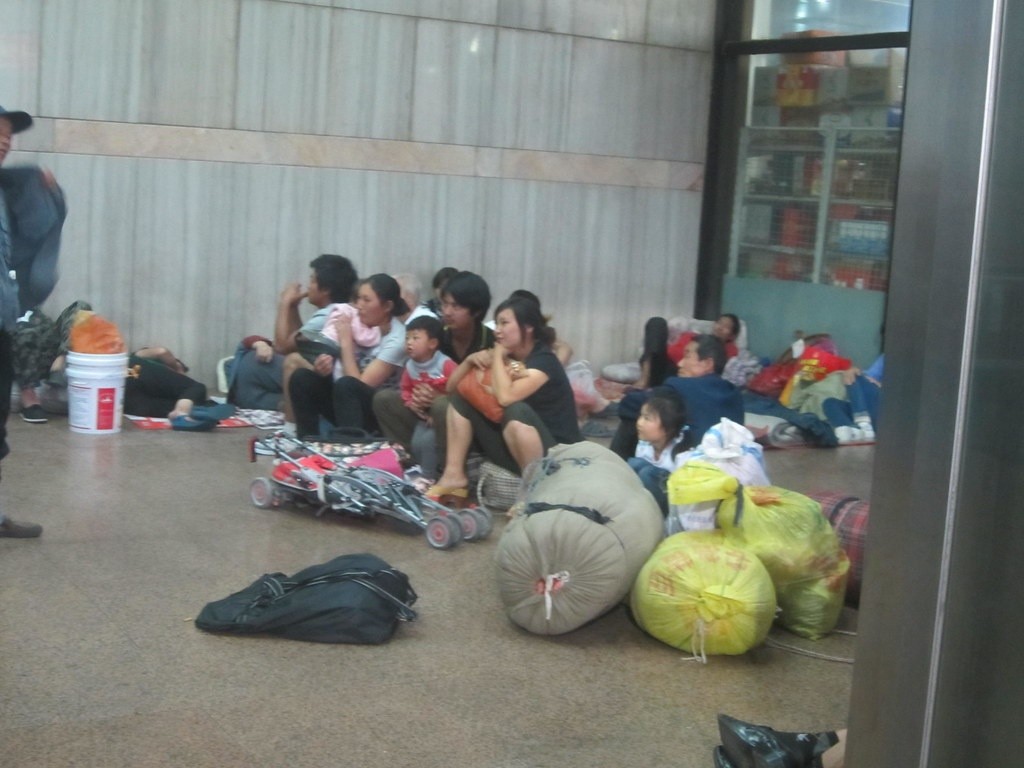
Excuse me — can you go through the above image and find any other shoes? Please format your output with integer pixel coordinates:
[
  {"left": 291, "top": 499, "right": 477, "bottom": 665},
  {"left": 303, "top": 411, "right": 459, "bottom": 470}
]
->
[
  {"left": 0, "top": 517, "right": 42, "bottom": 537},
  {"left": 172, "top": 415, "right": 215, "bottom": 429},
  {"left": 192, "top": 404, "right": 236, "bottom": 421},
  {"left": 20, "top": 404, "right": 47, "bottom": 422},
  {"left": 50, "top": 372, "right": 65, "bottom": 386},
  {"left": 255, "top": 439, "right": 277, "bottom": 455}
]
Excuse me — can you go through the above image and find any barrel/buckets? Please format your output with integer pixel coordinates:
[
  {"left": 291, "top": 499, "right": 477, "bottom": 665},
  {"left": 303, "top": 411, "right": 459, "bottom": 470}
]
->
[{"left": 64, "top": 346, "right": 129, "bottom": 435}]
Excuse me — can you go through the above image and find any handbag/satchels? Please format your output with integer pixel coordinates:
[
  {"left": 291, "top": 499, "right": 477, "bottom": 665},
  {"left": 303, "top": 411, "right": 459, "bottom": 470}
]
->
[
  {"left": 456, "top": 357, "right": 525, "bottom": 422},
  {"left": 302, "top": 426, "right": 415, "bottom": 471},
  {"left": 477, "top": 460, "right": 522, "bottom": 511},
  {"left": 805, "top": 486, "right": 869, "bottom": 604}
]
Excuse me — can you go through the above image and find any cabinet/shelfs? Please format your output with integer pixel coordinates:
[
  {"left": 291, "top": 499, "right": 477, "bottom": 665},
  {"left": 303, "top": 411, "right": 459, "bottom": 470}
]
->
[{"left": 728, "top": 69, "right": 911, "bottom": 376}]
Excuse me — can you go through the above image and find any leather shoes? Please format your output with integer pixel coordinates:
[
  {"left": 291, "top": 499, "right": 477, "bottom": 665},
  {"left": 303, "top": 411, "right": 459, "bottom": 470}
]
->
[
  {"left": 714, "top": 745, "right": 737, "bottom": 768},
  {"left": 718, "top": 713, "right": 799, "bottom": 768}
]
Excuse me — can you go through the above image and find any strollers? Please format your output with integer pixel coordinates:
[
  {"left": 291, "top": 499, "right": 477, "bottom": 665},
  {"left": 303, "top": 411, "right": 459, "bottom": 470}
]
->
[{"left": 245, "top": 430, "right": 495, "bottom": 551}]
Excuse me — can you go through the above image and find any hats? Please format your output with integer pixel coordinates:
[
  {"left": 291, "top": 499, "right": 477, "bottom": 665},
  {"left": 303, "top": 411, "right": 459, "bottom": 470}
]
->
[{"left": 0, "top": 106, "right": 33, "bottom": 133}]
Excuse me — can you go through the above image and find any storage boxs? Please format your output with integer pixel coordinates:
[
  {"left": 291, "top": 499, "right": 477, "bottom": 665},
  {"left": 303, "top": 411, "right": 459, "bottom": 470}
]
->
[
  {"left": 837, "top": 218, "right": 892, "bottom": 256},
  {"left": 773, "top": 63, "right": 841, "bottom": 107},
  {"left": 777, "top": 29, "right": 851, "bottom": 70}
]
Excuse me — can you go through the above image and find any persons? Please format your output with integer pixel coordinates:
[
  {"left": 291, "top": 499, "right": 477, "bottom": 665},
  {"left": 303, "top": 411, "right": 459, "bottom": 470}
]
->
[
  {"left": 11, "top": 301, "right": 92, "bottom": 422},
  {"left": 228, "top": 336, "right": 287, "bottom": 411},
  {"left": 274, "top": 253, "right": 622, "bottom": 502},
  {"left": 0, "top": 106, "right": 62, "bottom": 536},
  {"left": 123, "top": 345, "right": 238, "bottom": 431},
  {"left": 611, "top": 313, "right": 888, "bottom": 521}
]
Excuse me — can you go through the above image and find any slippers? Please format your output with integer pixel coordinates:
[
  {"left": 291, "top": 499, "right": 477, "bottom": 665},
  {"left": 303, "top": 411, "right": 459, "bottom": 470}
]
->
[
  {"left": 580, "top": 421, "right": 617, "bottom": 437},
  {"left": 589, "top": 401, "right": 619, "bottom": 416},
  {"left": 426, "top": 485, "right": 468, "bottom": 498}
]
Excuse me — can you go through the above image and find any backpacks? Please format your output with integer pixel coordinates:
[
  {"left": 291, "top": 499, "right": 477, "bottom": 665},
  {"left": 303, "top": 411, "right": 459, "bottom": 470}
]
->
[{"left": 194, "top": 552, "right": 417, "bottom": 644}]
